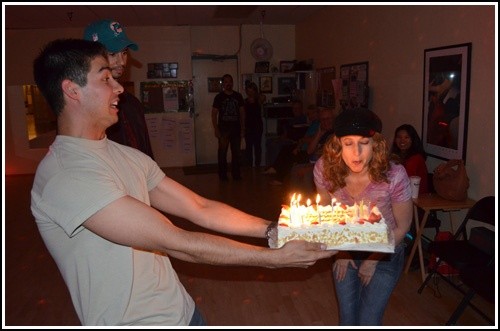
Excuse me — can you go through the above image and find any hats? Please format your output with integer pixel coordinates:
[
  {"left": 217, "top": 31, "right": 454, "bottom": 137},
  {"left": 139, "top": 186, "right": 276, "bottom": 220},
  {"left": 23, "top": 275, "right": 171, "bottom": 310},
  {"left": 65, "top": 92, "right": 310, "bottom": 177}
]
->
[
  {"left": 333, "top": 107, "right": 382, "bottom": 136},
  {"left": 84, "top": 19, "right": 139, "bottom": 53}
]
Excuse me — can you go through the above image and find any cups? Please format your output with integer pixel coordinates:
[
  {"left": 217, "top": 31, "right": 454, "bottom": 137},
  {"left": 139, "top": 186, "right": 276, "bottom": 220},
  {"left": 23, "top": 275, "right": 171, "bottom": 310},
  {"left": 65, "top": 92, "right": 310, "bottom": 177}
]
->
[{"left": 410, "top": 176, "right": 421, "bottom": 198}]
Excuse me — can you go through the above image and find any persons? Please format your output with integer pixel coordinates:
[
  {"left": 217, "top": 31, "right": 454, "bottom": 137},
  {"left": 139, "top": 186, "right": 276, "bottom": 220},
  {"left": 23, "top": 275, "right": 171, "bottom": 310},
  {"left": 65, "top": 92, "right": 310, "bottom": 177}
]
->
[
  {"left": 29, "top": 37, "right": 341, "bottom": 326},
  {"left": 242, "top": 81, "right": 264, "bottom": 175},
  {"left": 210, "top": 74, "right": 246, "bottom": 182},
  {"left": 310, "top": 107, "right": 415, "bottom": 325},
  {"left": 390, "top": 123, "right": 430, "bottom": 220},
  {"left": 264, "top": 101, "right": 335, "bottom": 186},
  {"left": 83, "top": 18, "right": 155, "bottom": 160}
]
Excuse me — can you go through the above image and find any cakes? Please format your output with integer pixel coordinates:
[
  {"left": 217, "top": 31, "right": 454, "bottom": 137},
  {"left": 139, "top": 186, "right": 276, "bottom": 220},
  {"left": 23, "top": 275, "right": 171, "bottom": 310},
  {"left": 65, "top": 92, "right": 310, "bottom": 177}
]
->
[{"left": 277, "top": 203, "right": 388, "bottom": 251}]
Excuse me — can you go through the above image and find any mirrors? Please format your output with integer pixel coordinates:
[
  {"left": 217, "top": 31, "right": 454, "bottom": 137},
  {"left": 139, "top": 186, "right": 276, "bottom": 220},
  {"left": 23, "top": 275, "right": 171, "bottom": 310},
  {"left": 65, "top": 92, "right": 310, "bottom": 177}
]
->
[{"left": 21, "top": 84, "right": 58, "bottom": 148}]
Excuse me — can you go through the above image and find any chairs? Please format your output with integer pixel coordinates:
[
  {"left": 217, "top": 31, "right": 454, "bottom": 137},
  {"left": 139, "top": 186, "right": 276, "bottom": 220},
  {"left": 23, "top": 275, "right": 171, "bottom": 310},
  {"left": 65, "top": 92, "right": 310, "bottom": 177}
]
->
[{"left": 417, "top": 196, "right": 494, "bottom": 325}]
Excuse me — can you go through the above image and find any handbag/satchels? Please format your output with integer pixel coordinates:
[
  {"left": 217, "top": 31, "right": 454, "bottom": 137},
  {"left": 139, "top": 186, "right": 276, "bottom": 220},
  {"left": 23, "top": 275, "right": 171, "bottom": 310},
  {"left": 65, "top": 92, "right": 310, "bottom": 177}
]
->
[
  {"left": 427, "top": 231, "right": 465, "bottom": 279},
  {"left": 433, "top": 159, "right": 469, "bottom": 201},
  {"left": 423, "top": 272, "right": 462, "bottom": 296}
]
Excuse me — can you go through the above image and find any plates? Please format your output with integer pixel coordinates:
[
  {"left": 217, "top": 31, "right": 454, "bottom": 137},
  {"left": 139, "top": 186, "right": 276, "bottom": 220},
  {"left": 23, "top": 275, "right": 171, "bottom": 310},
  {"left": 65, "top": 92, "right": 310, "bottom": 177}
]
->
[{"left": 267, "top": 228, "right": 396, "bottom": 254}]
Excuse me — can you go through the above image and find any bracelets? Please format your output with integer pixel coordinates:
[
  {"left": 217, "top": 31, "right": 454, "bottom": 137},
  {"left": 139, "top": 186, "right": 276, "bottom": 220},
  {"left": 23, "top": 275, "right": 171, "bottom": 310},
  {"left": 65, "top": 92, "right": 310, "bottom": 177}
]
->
[{"left": 265, "top": 222, "right": 276, "bottom": 241}]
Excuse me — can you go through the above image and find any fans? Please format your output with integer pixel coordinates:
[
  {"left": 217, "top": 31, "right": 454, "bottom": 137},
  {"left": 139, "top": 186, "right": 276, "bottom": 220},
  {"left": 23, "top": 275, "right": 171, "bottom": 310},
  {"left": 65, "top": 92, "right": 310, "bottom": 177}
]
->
[{"left": 251, "top": 38, "right": 273, "bottom": 62}]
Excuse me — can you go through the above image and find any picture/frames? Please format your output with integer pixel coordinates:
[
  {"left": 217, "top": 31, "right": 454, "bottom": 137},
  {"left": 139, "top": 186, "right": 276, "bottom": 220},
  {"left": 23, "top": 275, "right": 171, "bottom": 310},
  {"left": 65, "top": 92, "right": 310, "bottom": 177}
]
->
[
  {"left": 280, "top": 60, "right": 297, "bottom": 73},
  {"left": 319, "top": 66, "right": 337, "bottom": 109},
  {"left": 339, "top": 61, "right": 369, "bottom": 110},
  {"left": 254, "top": 62, "right": 269, "bottom": 73},
  {"left": 420, "top": 41, "right": 472, "bottom": 166}
]
